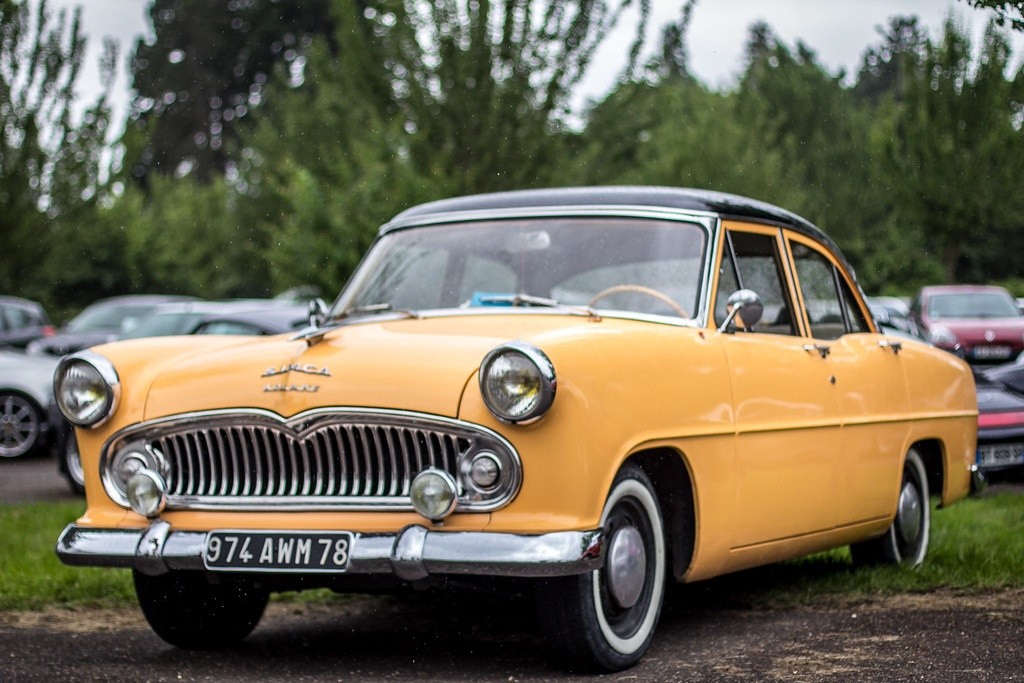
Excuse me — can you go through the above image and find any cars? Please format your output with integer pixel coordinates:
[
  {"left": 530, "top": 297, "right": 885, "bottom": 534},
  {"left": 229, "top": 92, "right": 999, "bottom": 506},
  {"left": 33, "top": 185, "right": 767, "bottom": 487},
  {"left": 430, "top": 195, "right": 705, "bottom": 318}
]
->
[
  {"left": 53, "top": 184, "right": 985, "bottom": 676},
  {"left": 0, "top": 285, "right": 329, "bottom": 463},
  {"left": 713, "top": 297, "right": 1024, "bottom": 473},
  {"left": 910, "top": 284, "right": 1024, "bottom": 366}
]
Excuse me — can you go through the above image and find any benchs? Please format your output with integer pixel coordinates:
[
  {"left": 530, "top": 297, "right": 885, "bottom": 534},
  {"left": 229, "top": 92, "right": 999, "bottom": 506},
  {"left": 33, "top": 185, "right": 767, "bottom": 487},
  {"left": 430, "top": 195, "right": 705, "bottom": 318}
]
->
[{"left": 756, "top": 324, "right": 859, "bottom": 339}]
